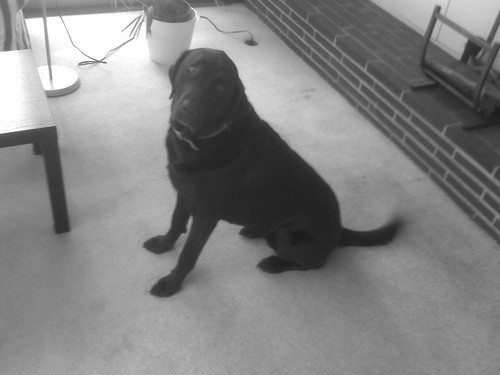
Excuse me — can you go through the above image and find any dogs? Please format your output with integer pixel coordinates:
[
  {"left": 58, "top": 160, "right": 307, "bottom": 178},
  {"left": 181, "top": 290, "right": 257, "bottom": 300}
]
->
[{"left": 141, "top": 48, "right": 405, "bottom": 298}]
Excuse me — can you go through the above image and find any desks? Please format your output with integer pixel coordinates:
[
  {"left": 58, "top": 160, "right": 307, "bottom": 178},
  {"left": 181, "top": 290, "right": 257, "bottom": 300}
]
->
[{"left": 0, "top": 49, "right": 71, "bottom": 235}]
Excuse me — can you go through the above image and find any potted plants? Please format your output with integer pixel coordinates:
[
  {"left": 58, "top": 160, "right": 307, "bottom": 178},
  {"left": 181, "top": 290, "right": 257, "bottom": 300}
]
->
[{"left": 121, "top": 0, "right": 198, "bottom": 39}]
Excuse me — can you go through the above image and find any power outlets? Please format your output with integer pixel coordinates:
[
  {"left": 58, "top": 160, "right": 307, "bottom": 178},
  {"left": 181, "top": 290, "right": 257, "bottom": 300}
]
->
[{"left": 245, "top": 39, "right": 259, "bottom": 46}]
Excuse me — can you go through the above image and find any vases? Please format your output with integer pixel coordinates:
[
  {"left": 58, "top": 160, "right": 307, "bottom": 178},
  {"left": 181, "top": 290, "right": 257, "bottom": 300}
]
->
[{"left": 145, "top": 6, "right": 198, "bottom": 66}]
